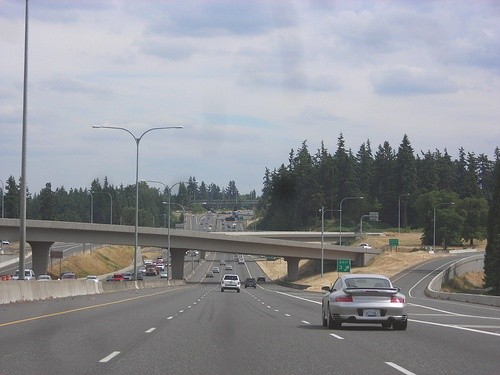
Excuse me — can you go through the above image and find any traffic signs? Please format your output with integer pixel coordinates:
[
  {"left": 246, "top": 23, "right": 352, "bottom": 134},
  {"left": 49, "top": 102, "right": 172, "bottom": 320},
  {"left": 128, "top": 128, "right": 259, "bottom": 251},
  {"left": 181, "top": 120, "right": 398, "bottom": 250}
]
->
[{"left": 337, "top": 259, "right": 351, "bottom": 273}]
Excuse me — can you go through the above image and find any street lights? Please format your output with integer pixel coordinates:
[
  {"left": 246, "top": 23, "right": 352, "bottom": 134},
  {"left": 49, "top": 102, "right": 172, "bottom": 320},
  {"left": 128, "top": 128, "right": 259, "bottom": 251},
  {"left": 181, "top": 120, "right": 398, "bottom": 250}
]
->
[
  {"left": 339, "top": 196, "right": 363, "bottom": 245},
  {"left": 398, "top": 194, "right": 411, "bottom": 246},
  {"left": 92, "top": 124, "right": 185, "bottom": 290},
  {"left": 92, "top": 191, "right": 112, "bottom": 225},
  {"left": 433, "top": 203, "right": 454, "bottom": 252},
  {"left": 147, "top": 180, "right": 199, "bottom": 287},
  {"left": 319, "top": 206, "right": 342, "bottom": 278}
]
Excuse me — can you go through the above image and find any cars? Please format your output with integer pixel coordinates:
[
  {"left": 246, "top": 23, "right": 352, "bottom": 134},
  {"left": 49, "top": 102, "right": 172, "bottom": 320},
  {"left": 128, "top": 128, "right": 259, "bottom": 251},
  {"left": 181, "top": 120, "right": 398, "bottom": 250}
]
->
[
  {"left": 244, "top": 278, "right": 257, "bottom": 289},
  {"left": 58, "top": 272, "right": 77, "bottom": 280},
  {"left": 358, "top": 243, "right": 371, "bottom": 249},
  {"left": 10, "top": 269, "right": 35, "bottom": 280},
  {"left": 184, "top": 214, "right": 245, "bottom": 278},
  {"left": 3, "top": 240, "right": 9, "bottom": 245},
  {"left": 256, "top": 277, "right": 265, "bottom": 284},
  {"left": 86, "top": 275, "right": 100, "bottom": 282},
  {"left": 321, "top": 274, "right": 409, "bottom": 331},
  {"left": 221, "top": 274, "right": 241, "bottom": 293},
  {"left": 37, "top": 275, "right": 52, "bottom": 281}
]
